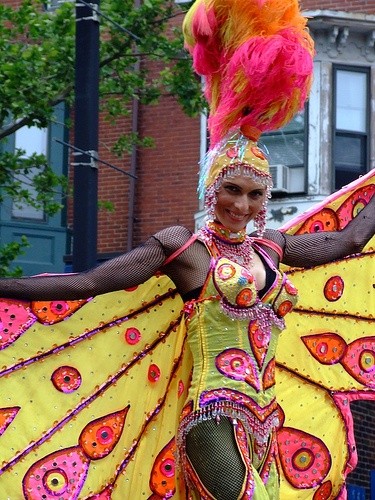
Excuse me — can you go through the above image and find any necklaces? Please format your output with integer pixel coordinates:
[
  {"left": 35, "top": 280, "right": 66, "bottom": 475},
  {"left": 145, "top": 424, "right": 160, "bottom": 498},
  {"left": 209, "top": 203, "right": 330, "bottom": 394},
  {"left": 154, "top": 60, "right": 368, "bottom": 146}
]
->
[{"left": 201, "top": 221, "right": 255, "bottom": 271}]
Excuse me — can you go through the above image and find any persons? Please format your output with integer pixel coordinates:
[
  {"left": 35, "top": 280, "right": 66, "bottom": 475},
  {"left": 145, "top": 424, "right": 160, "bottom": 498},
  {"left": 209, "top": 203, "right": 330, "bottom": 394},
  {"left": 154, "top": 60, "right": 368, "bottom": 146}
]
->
[{"left": 1, "top": 136, "right": 375, "bottom": 500}]
